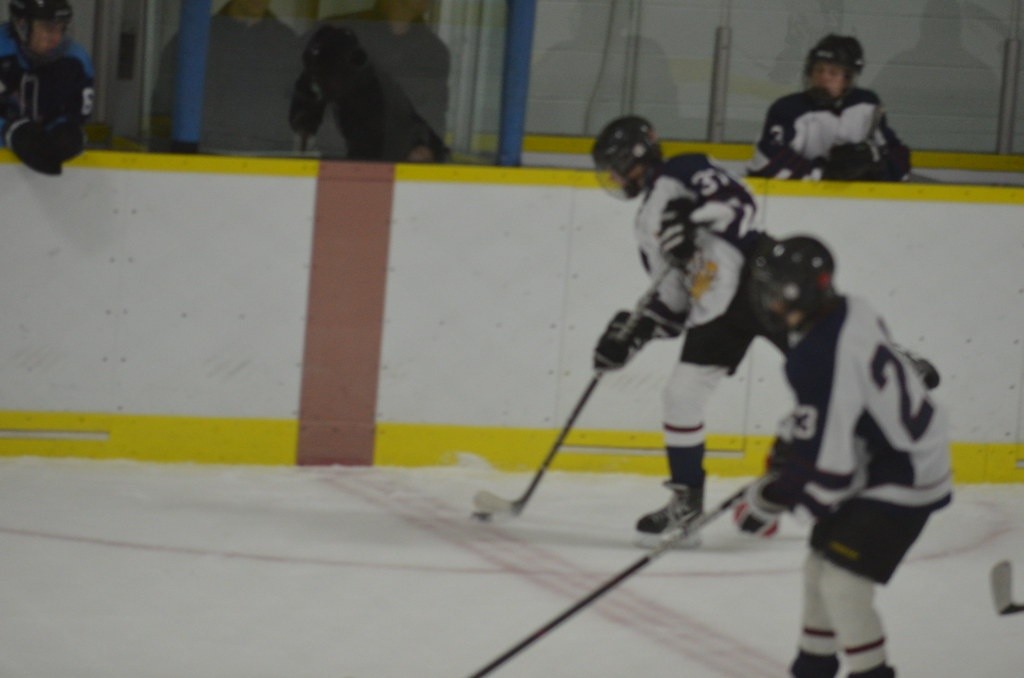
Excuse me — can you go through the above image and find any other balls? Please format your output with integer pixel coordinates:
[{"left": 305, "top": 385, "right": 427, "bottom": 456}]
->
[{"left": 472, "top": 511, "right": 493, "bottom": 521}]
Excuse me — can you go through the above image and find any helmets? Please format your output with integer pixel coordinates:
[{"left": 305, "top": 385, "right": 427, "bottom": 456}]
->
[
  {"left": 305, "top": 25, "right": 364, "bottom": 96},
  {"left": 10, "top": 0, "right": 73, "bottom": 46},
  {"left": 805, "top": 35, "right": 864, "bottom": 78},
  {"left": 752, "top": 237, "right": 835, "bottom": 335},
  {"left": 592, "top": 115, "right": 661, "bottom": 201}
]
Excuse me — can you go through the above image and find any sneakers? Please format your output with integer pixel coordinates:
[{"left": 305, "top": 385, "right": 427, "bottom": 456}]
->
[{"left": 637, "top": 472, "right": 705, "bottom": 535}]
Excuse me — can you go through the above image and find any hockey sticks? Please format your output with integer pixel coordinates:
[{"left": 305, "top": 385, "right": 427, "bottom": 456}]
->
[
  {"left": 466, "top": 478, "right": 764, "bottom": 678},
  {"left": 470, "top": 368, "right": 604, "bottom": 523},
  {"left": 987, "top": 559, "right": 1024, "bottom": 617}
]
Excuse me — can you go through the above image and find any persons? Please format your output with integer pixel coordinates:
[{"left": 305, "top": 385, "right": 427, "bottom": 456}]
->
[
  {"left": 733, "top": 235, "right": 952, "bottom": 678},
  {"left": 0, "top": 0, "right": 453, "bottom": 175},
  {"left": 745, "top": 35, "right": 913, "bottom": 182},
  {"left": 590, "top": 115, "right": 786, "bottom": 552}
]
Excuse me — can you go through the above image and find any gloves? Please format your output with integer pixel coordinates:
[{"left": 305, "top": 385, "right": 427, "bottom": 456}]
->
[
  {"left": 636, "top": 294, "right": 686, "bottom": 340},
  {"left": 595, "top": 312, "right": 646, "bottom": 369},
  {"left": 41, "top": 113, "right": 87, "bottom": 163},
  {"left": 3, "top": 115, "right": 62, "bottom": 176},
  {"left": 734, "top": 475, "right": 785, "bottom": 536}
]
